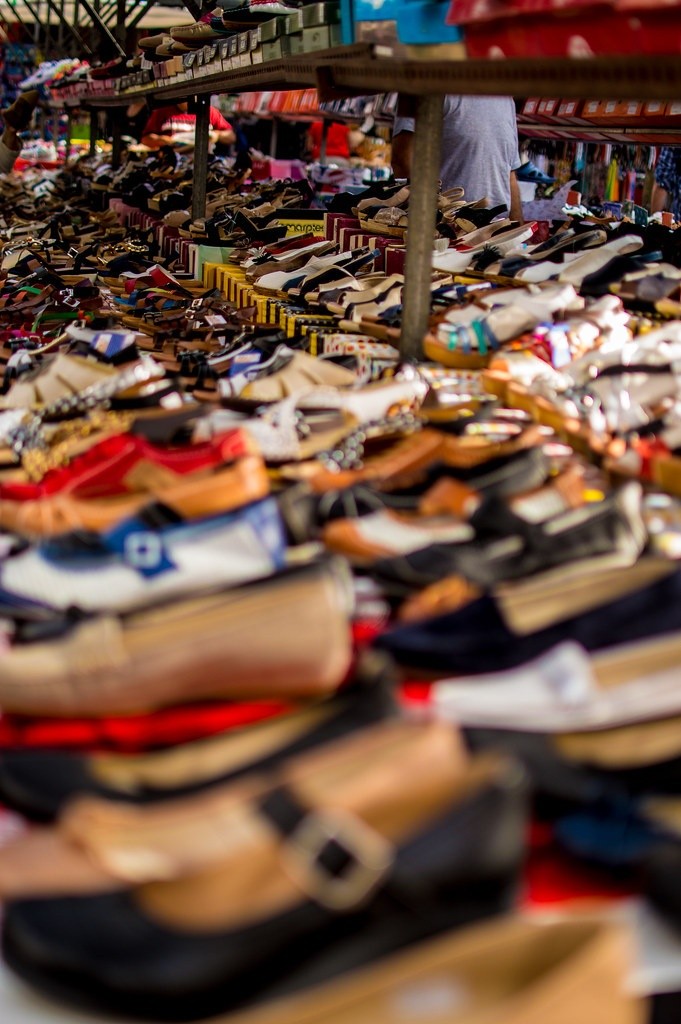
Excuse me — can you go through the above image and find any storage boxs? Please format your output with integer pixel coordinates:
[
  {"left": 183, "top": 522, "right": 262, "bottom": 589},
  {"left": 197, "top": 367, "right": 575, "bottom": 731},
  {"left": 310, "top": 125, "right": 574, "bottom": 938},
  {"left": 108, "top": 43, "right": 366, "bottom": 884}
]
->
[
  {"left": 48, "top": 0, "right": 681, "bottom": 145},
  {"left": 121, "top": 159, "right": 491, "bottom": 401}
]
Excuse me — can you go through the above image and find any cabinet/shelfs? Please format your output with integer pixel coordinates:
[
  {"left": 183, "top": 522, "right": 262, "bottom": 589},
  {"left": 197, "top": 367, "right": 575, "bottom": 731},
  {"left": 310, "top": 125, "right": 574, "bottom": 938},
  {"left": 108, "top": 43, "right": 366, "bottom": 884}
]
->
[{"left": 62, "top": 41, "right": 681, "bottom": 358}]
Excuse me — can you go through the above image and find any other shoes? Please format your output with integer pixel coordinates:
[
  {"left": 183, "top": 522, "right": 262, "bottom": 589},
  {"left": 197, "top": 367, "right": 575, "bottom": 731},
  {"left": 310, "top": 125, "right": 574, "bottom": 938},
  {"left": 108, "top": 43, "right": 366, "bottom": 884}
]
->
[
  {"left": 0, "top": 146, "right": 681, "bottom": 1024},
  {"left": 20, "top": 1, "right": 305, "bottom": 94}
]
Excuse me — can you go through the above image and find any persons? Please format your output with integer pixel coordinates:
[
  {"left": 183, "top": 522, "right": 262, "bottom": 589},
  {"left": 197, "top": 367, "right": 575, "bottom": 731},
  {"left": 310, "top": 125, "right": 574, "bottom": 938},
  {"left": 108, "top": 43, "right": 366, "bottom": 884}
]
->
[
  {"left": 306, "top": 118, "right": 366, "bottom": 171},
  {"left": 391, "top": 95, "right": 523, "bottom": 227},
  {"left": 0, "top": 40, "right": 39, "bottom": 173},
  {"left": 651, "top": 146, "right": 681, "bottom": 224},
  {"left": 141, "top": 96, "right": 236, "bottom": 158}
]
